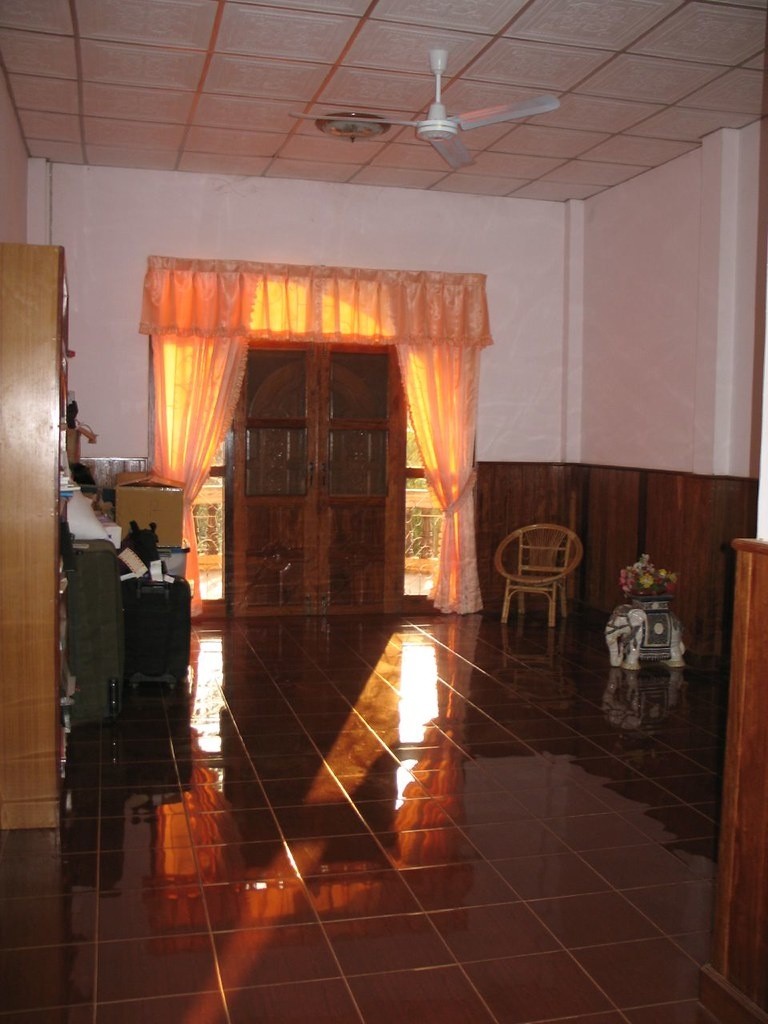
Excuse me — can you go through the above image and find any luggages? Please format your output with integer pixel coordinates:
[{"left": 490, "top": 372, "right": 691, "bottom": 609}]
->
[{"left": 122, "top": 574, "right": 191, "bottom": 690}]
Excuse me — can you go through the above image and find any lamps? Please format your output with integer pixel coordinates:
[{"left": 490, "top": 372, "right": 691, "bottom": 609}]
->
[{"left": 314, "top": 113, "right": 391, "bottom": 144}]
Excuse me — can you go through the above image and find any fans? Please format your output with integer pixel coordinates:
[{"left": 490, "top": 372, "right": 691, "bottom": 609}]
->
[{"left": 288, "top": 50, "right": 561, "bottom": 168}]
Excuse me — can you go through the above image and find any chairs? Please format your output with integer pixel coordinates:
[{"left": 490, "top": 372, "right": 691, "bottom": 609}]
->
[{"left": 494, "top": 524, "right": 584, "bottom": 627}]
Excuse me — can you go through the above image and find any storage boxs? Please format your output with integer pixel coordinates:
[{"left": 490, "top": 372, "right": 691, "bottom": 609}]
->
[
  {"left": 160, "top": 546, "right": 191, "bottom": 578},
  {"left": 115, "top": 470, "right": 186, "bottom": 548}
]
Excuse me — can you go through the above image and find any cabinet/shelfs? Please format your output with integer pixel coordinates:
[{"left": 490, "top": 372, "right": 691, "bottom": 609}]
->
[{"left": 0, "top": 241, "right": 70, "bottom": 830}]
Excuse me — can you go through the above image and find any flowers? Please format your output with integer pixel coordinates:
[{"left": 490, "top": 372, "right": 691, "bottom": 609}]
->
[{"left": 619, "top": 554, "right": 677, "bottom": 596}]
